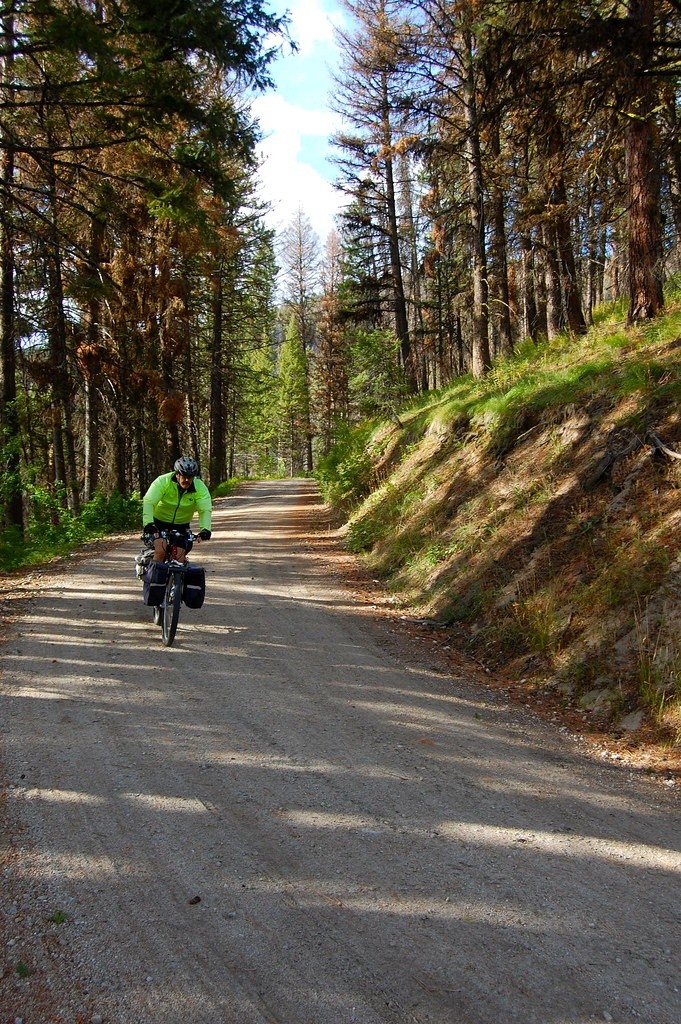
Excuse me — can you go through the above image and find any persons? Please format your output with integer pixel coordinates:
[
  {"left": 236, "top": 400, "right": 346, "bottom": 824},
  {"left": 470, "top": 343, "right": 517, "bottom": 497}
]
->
[{"left": 143, "top": 456, "right": 211, "bottom": 565}]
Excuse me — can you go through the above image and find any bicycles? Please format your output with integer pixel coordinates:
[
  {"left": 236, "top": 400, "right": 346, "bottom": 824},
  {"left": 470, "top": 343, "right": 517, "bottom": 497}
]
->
[{"left": 130, "top": 531, "right": 208, "bottom": 649}]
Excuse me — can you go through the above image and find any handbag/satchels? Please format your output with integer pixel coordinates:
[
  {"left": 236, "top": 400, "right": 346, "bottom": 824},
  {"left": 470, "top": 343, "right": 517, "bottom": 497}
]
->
[
  {"left": 134, "top": 549, "right": 156, "bottom": 580},
  {"left": 143, "top": 562, "right": 168, "bottom": 605},
  {"left": 185, "top": 563, "right": 205, "bottom": 609}
]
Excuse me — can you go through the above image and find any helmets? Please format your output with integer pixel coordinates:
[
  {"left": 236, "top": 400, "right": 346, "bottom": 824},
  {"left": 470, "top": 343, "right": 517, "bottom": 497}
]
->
[{"left": 174, "top": 456, "right": 198, "bottom": 477}]
